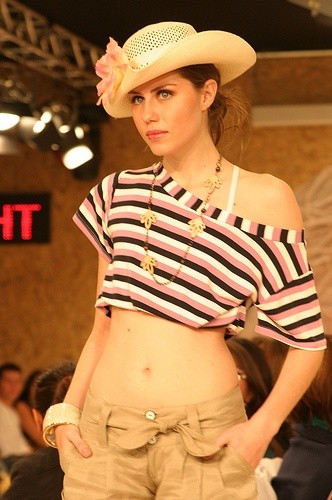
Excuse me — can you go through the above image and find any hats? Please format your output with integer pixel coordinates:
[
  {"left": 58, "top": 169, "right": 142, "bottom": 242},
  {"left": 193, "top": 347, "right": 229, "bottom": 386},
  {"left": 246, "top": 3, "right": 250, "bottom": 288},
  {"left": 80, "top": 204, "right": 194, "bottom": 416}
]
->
[{"left": 95, "top": 20, "right": 256, "bottom": 119}]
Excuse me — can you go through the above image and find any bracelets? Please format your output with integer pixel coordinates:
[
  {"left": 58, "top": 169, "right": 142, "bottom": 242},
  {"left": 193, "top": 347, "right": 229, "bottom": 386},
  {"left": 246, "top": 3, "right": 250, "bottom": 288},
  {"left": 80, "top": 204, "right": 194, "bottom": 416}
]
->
[{"left": 43, "top": 403, "right": 81, "bottom": 448}]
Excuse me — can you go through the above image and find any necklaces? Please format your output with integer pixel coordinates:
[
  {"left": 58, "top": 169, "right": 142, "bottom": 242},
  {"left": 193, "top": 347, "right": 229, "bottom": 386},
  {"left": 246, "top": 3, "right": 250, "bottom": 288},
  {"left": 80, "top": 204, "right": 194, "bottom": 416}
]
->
[{"left": 140, "top": 152, "right": 224, "bottom": 285}]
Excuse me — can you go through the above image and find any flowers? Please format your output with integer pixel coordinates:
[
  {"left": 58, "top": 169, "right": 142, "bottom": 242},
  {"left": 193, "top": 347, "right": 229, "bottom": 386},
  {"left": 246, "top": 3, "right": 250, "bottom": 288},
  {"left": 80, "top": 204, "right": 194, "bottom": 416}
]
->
[{"left": 93, "top": 37, "right": 126, "bottom": 107}]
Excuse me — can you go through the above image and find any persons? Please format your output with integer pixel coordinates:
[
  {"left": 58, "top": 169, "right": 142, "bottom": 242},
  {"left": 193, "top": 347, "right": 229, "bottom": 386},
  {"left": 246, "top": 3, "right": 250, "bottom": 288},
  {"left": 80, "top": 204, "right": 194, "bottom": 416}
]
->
[
  {"left": 42, "top": 22, "right": 327, "bottom": 500},
  {"left": 0, "top": 334, "right": 332, "bottom": 500}
]
